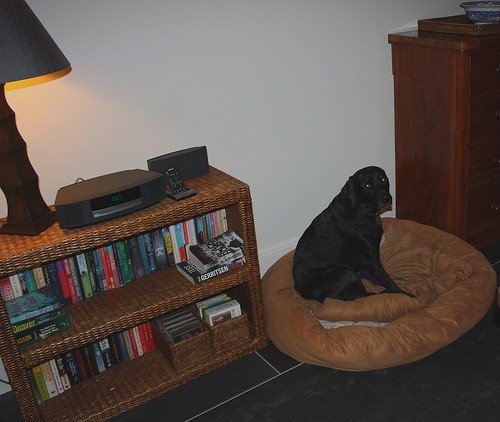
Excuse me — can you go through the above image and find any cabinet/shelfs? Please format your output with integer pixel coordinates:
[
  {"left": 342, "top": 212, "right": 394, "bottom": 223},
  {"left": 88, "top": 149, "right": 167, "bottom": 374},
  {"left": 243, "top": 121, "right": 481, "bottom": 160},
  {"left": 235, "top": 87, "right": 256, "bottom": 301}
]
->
[
  {"left": 388, "top": 30, "right": 500, "bottom": 251},
  {"left": 0, "top": 165, "right": 268, "bottom": 422}
]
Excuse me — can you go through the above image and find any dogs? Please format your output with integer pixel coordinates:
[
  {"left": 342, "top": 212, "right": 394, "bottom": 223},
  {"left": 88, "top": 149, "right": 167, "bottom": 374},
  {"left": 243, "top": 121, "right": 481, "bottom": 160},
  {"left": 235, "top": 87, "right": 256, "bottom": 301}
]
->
[{"left": 292, "top": 166, "right": 417, "bottom": 304}]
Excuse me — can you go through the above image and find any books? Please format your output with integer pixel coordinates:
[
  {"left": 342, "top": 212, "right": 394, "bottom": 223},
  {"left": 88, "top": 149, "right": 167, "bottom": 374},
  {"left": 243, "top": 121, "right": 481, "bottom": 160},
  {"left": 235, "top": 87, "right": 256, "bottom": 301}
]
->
[
  {"left": 176, "top": 257, "right": 250, "bottom": 285},
  {"left": 0, "top": 209, "right": 241, "bottom": 404},
  {"left": 188, "top": 229, "right": 245, "bottom": 273}
]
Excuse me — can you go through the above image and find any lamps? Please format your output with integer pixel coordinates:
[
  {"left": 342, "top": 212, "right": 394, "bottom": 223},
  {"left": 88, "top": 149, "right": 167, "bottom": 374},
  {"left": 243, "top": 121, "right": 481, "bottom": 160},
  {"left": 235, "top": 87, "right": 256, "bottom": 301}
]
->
[{"left": 0, "top": 0, "right": 72, "bottom": 238}]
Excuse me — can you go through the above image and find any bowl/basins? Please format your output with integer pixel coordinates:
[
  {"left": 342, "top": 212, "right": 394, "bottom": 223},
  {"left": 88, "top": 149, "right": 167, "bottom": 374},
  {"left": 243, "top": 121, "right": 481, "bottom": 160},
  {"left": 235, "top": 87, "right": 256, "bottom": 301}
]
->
[{"left": 459, "top": 1, "right": 500, "bottom": 23}]
